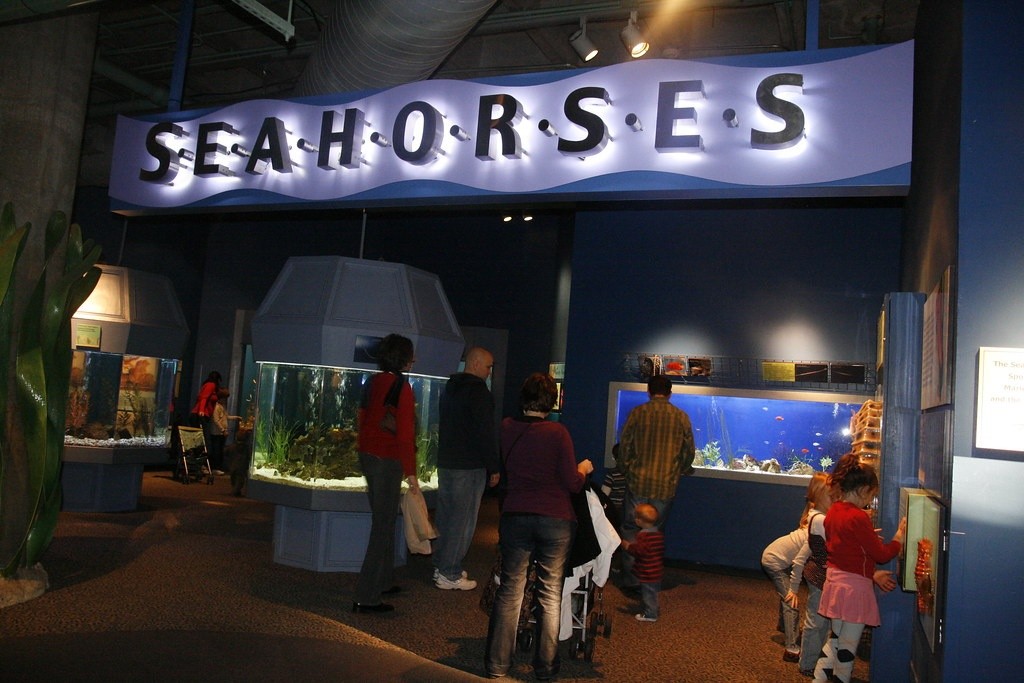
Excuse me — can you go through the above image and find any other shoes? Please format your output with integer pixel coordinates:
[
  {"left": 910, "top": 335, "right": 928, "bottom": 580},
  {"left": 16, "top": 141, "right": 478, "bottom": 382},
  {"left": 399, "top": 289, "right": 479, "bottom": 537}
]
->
[
  {"left": 538, "top": 660, "right": 560, "bottom": 679},
  {"left": 784, "top": 649, "right": 801, "bottom": 662},
  {"left": 800, "top": 666, "right": 832, "bottom": 679},
  {"left": 370, "top": 585, "right": 401, "bottom": 594},
  {"left": 212, "top": 469, "right": 224, "bottom": 475},
  {"left": 201, "top": 465, "right": 209, "bottom": 475},
  {"left": 353, "top": 603, "right": 394, "bottom": 615},
  {"left": 635, "top": 613, "right": 656, "bottom": 622}
]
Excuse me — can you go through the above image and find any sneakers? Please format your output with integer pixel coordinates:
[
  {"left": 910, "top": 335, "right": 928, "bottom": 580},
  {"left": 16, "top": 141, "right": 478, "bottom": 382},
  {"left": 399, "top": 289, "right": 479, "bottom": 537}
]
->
[
  {"left": 435, "top": 573, "right": 477, "bottom": 591},
  {"left": 433, "top": 568, "right": 468, "bottom": 581}
]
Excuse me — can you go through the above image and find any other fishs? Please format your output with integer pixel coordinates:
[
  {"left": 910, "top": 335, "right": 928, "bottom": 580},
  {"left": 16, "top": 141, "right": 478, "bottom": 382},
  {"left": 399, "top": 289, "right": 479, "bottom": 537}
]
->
[
  {"left": 776, "top": 416, "right": 784, "bottom": 421},
  {"left": 666, "top": 362, "right": 685, "bottom": 371},
  {"left": 802, "top": 449, "right": 809, "bottom": 453},
  {"left": 816, "top": 433, "right": 822, "bottom": 437},
  {"left": 813, "top": 442, "right": 824, "bottom": 451}
]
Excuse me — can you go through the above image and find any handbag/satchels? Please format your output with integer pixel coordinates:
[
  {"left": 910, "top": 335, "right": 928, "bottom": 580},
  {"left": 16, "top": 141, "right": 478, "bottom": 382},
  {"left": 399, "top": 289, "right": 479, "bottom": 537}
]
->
[
  {"left": 480, "top": 552, "right": 536, "bottom": 631},
  {"left": 402, "top": 484, "right": 436, "bottom": 555},
  {"left": 498, "top": 466, "right": 507, "bottom": 512}
]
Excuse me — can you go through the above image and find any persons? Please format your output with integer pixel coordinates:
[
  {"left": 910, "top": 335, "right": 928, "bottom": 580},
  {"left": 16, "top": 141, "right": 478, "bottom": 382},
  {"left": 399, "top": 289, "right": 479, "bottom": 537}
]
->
[
  {"left": 811, "top": 454, "right": 906, "bottom": 683},
  {"left": 209, "top": 387, "right": 243, "bottom": 474},
  {"left": 356, "top": 334, "right": 419, "bottom": 612},
  {"left": 622, "top": 504, "right": 664, "bottom": 621},
  {"left": 761, "top": 525, "right": 810, "bottom": 662},
  {"left": 601, "top": 444, "right": 625, "bottom": 573},
  {"left": 618, "top": 375, "right": 695, "bottom": 591},
  {"left": 798, "top": 471, "right": 884, "bottom": 676},
  {"left": 190, "top": 371, "right": 222, "bottom": 474},
  {"left": 433, "top": 347, "right": 500, "bottom": 591},
  {"left": 483, "top": 371, "right": 594, "bottom": 679}
]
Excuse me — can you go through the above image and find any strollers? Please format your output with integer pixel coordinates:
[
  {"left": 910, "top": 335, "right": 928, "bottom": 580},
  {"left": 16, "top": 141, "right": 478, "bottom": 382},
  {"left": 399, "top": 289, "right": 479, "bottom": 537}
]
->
[
  {"left": 517, "top": 477, "right": 613, "bottom": 665},
  {"left": 173, "top": 412, "right": 215, "bottom": 485}
]
